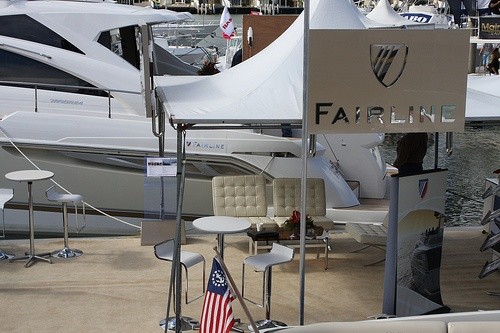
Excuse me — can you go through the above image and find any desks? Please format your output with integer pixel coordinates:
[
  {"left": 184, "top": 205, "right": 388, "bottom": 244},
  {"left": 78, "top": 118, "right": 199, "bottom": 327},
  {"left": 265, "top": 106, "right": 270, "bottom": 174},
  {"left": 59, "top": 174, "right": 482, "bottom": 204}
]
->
[
  {"left": 5, "top": 169, "right": 54, "bottom": 266},
  {"left": 193, "top": 216, "right": 250, "bottom": 258},
  {"left": 246, "top": 229, "right": 328, "bottom": 271}
]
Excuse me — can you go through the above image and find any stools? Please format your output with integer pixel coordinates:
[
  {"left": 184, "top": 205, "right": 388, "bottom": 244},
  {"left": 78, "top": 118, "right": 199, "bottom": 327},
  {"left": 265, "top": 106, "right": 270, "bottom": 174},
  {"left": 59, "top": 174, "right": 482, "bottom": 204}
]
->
[
  {"left": 242, "top": 242, "right": 294, "bottom": 332},
  {"left": 0, "top": 188, "right": 14, "bottom": 261},
  {"left": 154, "top": 239, "right": 206, "bottom": 332},
  {"left": 45, "top": 185, "right": 87, "bottom": 259}
]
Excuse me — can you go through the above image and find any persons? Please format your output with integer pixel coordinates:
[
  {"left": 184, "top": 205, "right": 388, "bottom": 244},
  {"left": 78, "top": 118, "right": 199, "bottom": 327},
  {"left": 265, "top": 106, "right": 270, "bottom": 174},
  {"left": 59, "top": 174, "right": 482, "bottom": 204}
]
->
[
  {"left": 448, "top": 0, "right": 500, "bottom": 28},
  {"left": 389, "top": 132, "right": 429, "bottom": 176},
  {"left": 197, "top": 53, "right": 220, "bottom": 76},
  {"left": 480, "top": 42, "right": 500, "bottom": 75}
]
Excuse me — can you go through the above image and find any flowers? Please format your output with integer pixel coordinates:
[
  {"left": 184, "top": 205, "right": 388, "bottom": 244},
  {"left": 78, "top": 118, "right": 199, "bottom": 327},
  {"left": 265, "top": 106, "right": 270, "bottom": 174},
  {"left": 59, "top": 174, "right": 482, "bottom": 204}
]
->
[{"left": 278, "top": 210, "right": 318, "bottom": 239}]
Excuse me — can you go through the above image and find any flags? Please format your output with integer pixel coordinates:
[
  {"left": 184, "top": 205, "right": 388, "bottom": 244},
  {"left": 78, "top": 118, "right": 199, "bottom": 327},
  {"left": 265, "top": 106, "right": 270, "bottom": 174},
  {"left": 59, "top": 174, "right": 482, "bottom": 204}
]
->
[
  {"left": 199, "top": 254, "right": 238, "bottom": 333},
  {"left": 219, "top": 7, "right": 235, "bottom": 39}
]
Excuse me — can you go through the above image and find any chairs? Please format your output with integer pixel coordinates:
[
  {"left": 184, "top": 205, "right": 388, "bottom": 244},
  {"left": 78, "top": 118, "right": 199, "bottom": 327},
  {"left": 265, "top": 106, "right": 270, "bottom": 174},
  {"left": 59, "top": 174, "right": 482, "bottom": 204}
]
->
[
  {"left": 212, "top": 175, "right": 278, "bottom": 230},
  {"left": 345, "top": 211, "right": 388, "bottom": 266},
  {"left": 269, "top": 179, "right": 335, "bottom": 232}
]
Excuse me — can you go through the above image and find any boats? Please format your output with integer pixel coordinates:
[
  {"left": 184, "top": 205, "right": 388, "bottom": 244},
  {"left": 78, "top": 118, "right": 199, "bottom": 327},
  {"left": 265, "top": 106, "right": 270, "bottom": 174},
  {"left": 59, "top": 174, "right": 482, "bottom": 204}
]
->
[{"left": 0, "top": 1, "right": 500, "bottom": 240}]
